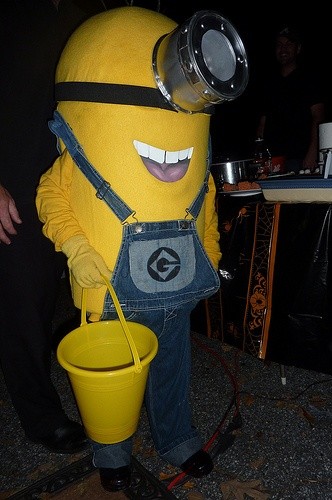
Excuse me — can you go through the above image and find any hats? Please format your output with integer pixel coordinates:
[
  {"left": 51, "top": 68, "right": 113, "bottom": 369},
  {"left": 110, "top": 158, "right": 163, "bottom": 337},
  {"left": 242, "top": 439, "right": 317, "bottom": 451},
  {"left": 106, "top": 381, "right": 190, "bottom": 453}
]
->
[{"left": 277, "top": 22, "right": 301, "bottom": 44}]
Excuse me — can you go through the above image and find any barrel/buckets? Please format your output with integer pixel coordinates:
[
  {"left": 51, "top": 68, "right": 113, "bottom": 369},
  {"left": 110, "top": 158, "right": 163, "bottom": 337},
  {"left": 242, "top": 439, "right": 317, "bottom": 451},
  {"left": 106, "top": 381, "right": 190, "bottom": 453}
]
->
[
  {"left": 57, "top": 274, "right": 158, "bottom": 444},
  {"left": 210, "top": 159, "right": 255, "bottom": 191}
]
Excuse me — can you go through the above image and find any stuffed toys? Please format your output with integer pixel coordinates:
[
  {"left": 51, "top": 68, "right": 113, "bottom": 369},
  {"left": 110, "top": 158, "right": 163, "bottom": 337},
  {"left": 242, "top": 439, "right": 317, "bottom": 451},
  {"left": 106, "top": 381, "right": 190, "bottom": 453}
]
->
[{"left": 35, "top": 6, "right": 250, "bottom": 490}]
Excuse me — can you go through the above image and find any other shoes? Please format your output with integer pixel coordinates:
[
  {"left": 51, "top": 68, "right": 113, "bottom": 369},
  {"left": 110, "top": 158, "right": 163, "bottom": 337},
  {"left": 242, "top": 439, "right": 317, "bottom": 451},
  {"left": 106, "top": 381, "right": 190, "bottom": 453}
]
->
[{"left": 41, "top": 423, "right": 85, "bottom": 454}]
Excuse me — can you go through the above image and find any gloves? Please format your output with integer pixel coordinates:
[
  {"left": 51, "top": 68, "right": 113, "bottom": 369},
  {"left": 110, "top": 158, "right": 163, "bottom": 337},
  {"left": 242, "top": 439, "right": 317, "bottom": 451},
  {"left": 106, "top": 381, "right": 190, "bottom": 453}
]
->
[{"left": 65, "top": 242, "right": 115, "bottom": 289}]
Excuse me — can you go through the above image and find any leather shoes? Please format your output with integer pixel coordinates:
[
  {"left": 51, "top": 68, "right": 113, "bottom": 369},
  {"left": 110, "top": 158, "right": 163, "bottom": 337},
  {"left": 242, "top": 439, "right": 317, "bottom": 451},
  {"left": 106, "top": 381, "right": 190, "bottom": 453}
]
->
[
  {"left": 181, "top": 450, "right": 213, "bottom": 478},
  {"left": 100, "top": 468, "right": 131, "bottom": 492}
]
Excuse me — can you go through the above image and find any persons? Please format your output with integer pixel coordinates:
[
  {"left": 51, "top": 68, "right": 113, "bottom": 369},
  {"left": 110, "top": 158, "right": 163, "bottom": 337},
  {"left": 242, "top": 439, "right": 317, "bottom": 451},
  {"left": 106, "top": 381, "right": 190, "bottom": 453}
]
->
[{"left": 0, "top": 186, "right": 82, "bottom": 453}]
